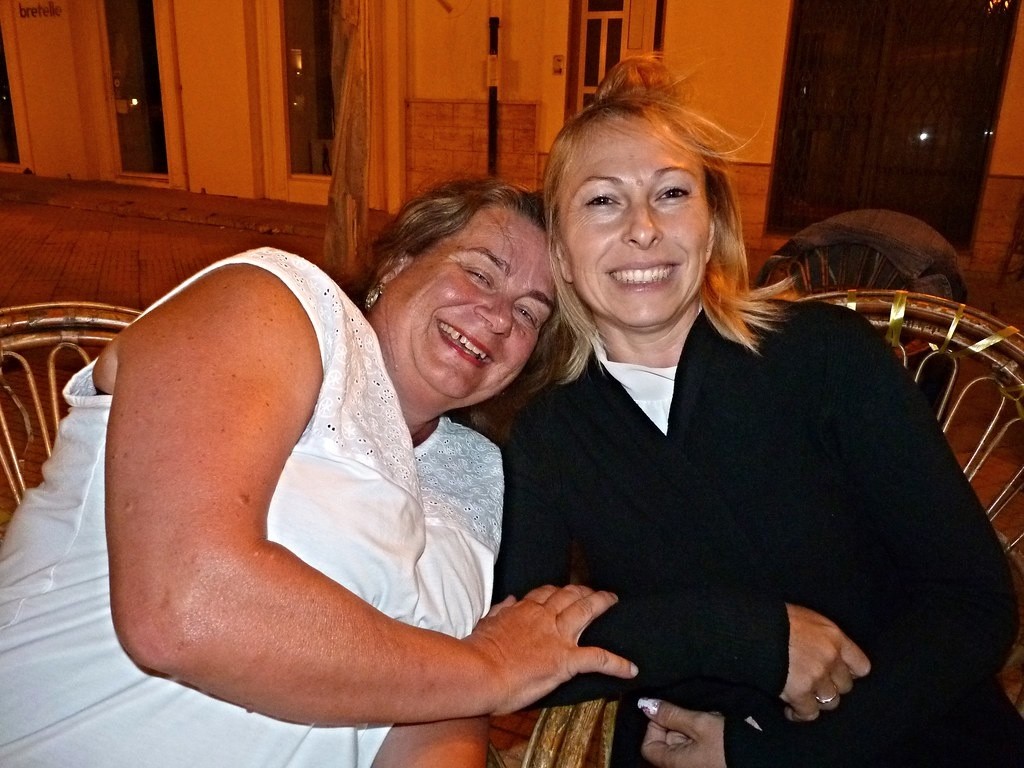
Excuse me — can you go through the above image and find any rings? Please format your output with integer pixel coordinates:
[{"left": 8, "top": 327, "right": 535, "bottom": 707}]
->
[{"left": 816, "top": 685, "right": 838, "bottom": 703}]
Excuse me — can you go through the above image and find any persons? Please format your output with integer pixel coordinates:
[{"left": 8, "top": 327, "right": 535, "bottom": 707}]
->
[
  {"left": 490, "top": 55, "right": 1024, "bottom": 768},
  {"left": 0, "top": 173, "right": 639, "bottom": 768}
]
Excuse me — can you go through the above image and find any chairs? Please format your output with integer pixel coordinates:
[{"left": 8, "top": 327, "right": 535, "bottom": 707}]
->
[{"left": 0, "top": 207, "right": 1024, "bottom": 768}]
